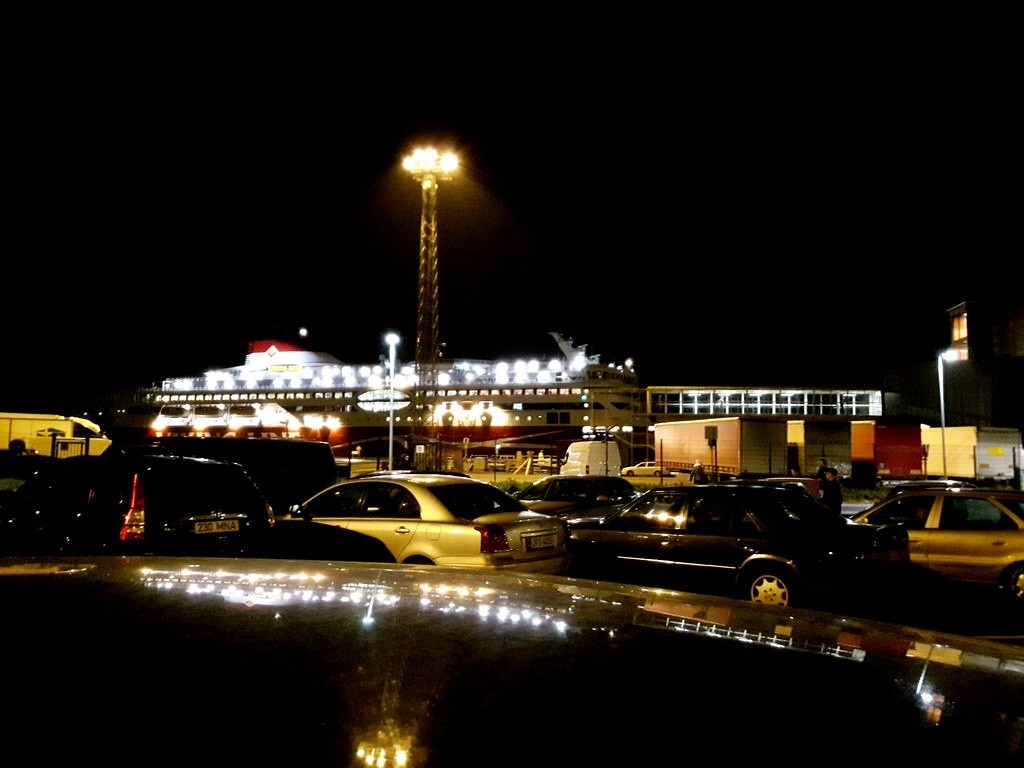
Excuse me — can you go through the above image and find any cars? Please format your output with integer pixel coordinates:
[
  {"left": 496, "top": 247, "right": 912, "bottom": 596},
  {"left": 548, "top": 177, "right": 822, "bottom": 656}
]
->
[
  {"left": 848, "top": 487, "right": 1024, "bottom": 605},
  {"left": 273, "top": 473, "right": 565, "bottom": 575},
  {"left": 0, "top": 454, "right": 274, "bottom": 558},
  {"left": 514, "top": 474, "right": 638, "bottom": 522},
  {"left": 886, "top": 481, "right": 979, "bottom": 498},
  {"left": 566, "top": 484, "right": 910, "bottom": 610},
  {"left": 622, "top": 462, "right": 670, "bottom": 477}
]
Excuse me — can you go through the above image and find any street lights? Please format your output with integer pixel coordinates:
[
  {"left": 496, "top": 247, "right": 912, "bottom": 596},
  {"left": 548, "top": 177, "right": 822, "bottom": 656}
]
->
[
  {"left": 938, "top": 352, "right": 957, "bottom": 478},
  {"left": 384, "top": 333, "right": 399, "bottom": 469},
  {"left": 398, "top": 145, "right": 459, "bottom": 472}
]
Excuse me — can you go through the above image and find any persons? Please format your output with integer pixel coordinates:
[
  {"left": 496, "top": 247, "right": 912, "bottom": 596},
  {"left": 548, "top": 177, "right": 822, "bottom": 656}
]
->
[
  {"left": 820, "top": 469, "right": 843, "bottom": 513},
  {"left": 538, "top": 450, "right": 545, "bottom": 464},
  {"left": 909, "top": 504, "right": 930, "bottom": 529},
  {"left": 805, "top": 458, "right": 828, "bottom": 478},
  {"left": 690, "top": 462, "right": 708, "bottom": 485},
  {"left": 790, "top": 465, "right": 803, "bottom": 477}
]
